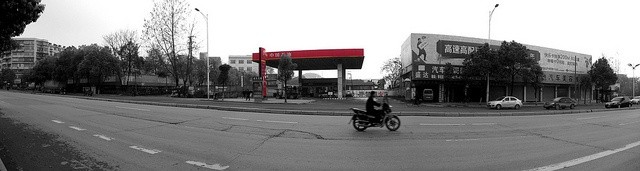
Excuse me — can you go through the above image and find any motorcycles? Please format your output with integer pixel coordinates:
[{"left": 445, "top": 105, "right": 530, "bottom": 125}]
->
[{"left": 348, "top": 103, "right": 401, "bottom": 132}]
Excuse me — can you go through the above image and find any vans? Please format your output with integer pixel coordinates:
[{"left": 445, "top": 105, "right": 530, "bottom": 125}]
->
[{"left": 423, "top": 89, "right": 434, "bottom": 102}]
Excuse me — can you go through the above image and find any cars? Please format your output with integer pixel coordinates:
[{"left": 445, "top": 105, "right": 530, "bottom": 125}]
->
[
  {"left": 604, "top": 97, "right": 632, "bottom": 109},
  {"left": 544, "top": 97, "right": 576, "bottom": 110},
  {"left": 487, "top": 96, "right": 522, "bottom": 111},
  {"left": 629, "top": 96, "right": 640, "bottom": 105}
]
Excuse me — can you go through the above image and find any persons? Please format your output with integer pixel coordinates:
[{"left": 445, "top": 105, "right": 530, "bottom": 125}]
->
[
  {"left": 417, "top": 38, "right": 427, "bottom": 61},
  {"left": 366, "top": 91, "right": 383, "bottom": 125}
]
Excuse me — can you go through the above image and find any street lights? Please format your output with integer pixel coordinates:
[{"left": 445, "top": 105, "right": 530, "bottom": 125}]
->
[
  {"left": 627, "top": 62, "right": 640, "bottom": 100},
  {"left": 486, "top": 4, "right": 499, "bottom": 109},
  {"left": 194, "top": 8, "right": 210, "bottom": 101}
]
